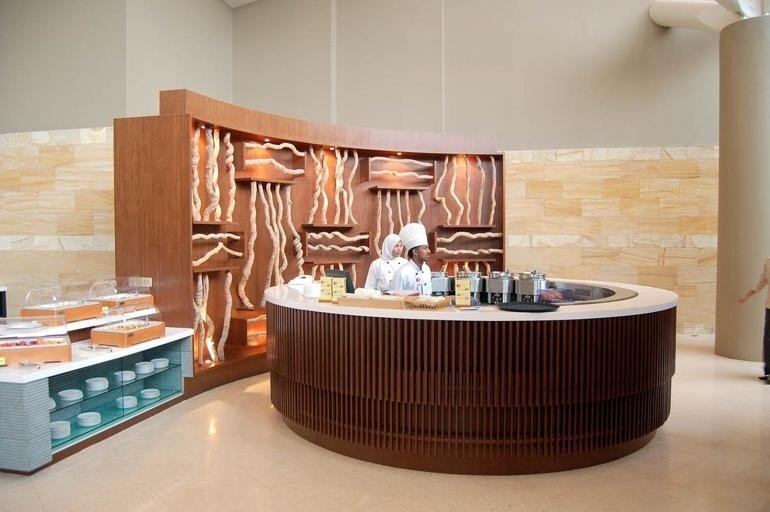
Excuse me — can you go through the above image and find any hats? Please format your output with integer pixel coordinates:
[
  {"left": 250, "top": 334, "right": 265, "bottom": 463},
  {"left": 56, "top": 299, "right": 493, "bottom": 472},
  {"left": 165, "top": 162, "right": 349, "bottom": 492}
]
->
[{"left": 399, "top": 223, "right": 428, "bottom": 255}]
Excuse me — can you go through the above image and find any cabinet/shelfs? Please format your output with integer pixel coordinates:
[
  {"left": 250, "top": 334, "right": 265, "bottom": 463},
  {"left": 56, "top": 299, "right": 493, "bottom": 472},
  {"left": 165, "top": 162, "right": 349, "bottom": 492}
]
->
[
  {"left": 114, "top": 89, "right": 508, "bottom": 398},
  {"left": 0, "top": 276, "right": 195, "bottom": 475}
]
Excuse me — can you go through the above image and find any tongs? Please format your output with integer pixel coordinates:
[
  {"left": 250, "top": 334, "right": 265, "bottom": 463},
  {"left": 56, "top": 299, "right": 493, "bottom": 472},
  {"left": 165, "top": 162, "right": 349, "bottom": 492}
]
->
[
  {"left": 87, "top": 345, "right": 113, "bottom": 353},
  {"left": 17, "top": 360, "right": 41, "bottom": 370}
]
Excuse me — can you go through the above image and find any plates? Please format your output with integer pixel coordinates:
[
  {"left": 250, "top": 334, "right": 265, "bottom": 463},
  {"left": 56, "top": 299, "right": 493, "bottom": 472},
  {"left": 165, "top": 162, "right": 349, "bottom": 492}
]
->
[
  {"left": 8, "top": 321, "right": 43, "bottom": 330},
  {"left": 116, "top": 395, "right": 138, "bottom": 409},
  {"left": 84, "top": 377, "right": 109, "bottom": 392},
  {"left": 76, "top": 411, "right": 102, "bottom": 426},
  {"left": 50, "top": 420, "right": 72, "bottom": 440},
  {"left": 141, "top": 388, "right": 160, "bottom": 399},
  {"left": 135, "top": 358, "right": 169, "bottom": 374},
  {"left": 48, "top": 397, "right": 57, "bottom": 409},
  {"left": 57, "top": 389, "right": 84, "bottom": 401},
  {"left": 114, "top": 370, "right": 136, "bottom": 381}
]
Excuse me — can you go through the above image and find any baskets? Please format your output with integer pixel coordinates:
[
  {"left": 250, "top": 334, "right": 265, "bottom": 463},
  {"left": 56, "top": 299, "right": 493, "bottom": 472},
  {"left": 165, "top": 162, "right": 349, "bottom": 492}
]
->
[{"left": 404, "top": 296, "right": 451, "bottom": 311}]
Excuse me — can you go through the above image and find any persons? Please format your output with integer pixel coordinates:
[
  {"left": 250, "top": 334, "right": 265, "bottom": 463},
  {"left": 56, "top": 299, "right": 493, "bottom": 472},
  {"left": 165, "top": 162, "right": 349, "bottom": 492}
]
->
[
  {"left": 736, "top": 256, "right": 770, "bottom": 385},
  {"left": 390, "top": 220, "right": 434, "bottom": 295},
  {"left": 364, "top": 233, "right": 409, "bottom": 294}
]
